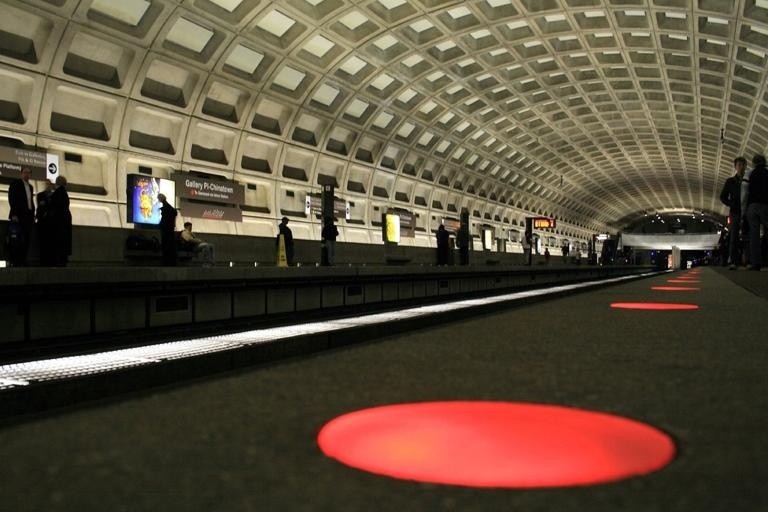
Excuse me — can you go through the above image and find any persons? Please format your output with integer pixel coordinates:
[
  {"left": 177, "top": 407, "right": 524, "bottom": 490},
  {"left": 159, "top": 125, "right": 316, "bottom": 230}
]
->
[
  {"left": 690, "top": 245, "right": 718, "bottom": 266},
  {"left": 178, "top": 221, "right": 214, "bottom": 264},
  {"left": 571, "top": 247, "right": 575, "bottom": 257},
  {"left": 322, "top": 219, "right": 339, "bottom": 264},
  {"left": 740, "top": 154, "right": 768, "bottom": 272},
  {"left": 157, "top": 193, "right": 178, "bottom": 259},
  {"left": 457, "top": 223, "right": 469, "bottom": 266},
  {"left": 8, "top": 166, "right": 72, "bottom": 264},
  {"left": 720, "top": 157, "right": 752, "bottom": 270},
  {"left": 278, "top": 217, "right": 294, "bottom": 264},
  {"left": 522, "top": 230, "right": 532, "bottom": 265},
  {"left": 436, "top": 224, "right": 449, "bottom": 265},
  {"left": 575, "top": 248, "right": 581, "bottom": 265},
  {"left": 545, "top": 248, "right": 549, "bottom": 263},
  {"left": 562, "top": 243, "right": 568, "bottom": 265}
]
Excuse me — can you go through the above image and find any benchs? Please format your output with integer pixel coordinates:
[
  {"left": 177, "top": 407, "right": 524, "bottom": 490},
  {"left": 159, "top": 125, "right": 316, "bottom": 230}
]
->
[
  {"left": 485, "top": 259, "right": 502, "bottom": 267},
  {"left": 385, "top": 255, "right": 411, "bottom": 266},
  {"left": 122, "top": 249, "right": 193, "bottom": 267}
]
